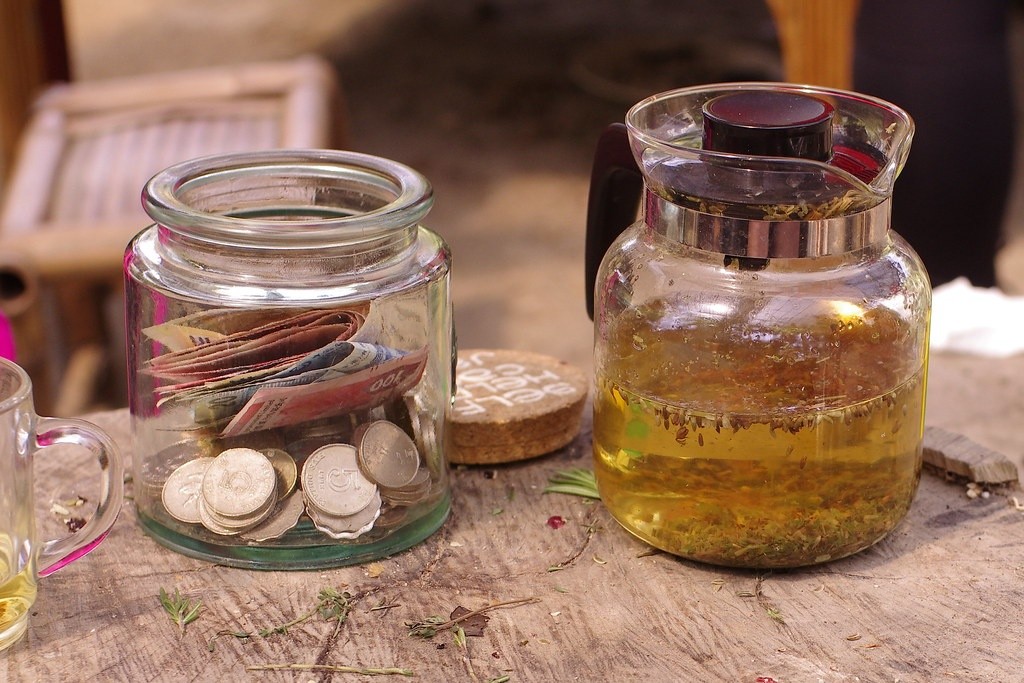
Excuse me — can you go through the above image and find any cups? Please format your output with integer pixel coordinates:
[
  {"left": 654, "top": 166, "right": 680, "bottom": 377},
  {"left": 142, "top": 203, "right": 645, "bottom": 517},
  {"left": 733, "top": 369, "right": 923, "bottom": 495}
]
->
[{"left": 0, "top": 356, "right": 125, "bottom": 660}]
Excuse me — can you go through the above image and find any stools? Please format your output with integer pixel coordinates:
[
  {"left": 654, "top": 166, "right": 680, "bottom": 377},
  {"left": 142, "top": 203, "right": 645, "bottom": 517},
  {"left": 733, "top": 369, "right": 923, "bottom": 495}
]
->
[{"left": 0, "top": 55, "right": 341, "bottom": 417}]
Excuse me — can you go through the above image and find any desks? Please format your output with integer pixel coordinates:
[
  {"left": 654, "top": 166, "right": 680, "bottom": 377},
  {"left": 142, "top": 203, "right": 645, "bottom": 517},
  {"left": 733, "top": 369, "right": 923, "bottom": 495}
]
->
[{"left": 0, "top": 352, "right": 1023, "bottom": 683}]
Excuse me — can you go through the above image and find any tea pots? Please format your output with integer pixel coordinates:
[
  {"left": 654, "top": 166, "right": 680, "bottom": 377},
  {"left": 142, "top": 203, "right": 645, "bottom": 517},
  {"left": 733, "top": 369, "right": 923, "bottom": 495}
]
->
[{"left": 584, "top": 81, "right": 932, "bottom": 570}]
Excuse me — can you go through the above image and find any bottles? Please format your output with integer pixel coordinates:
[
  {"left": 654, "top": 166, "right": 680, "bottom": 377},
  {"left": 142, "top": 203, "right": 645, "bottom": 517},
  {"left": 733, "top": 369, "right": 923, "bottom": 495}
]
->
[{"left": 123, "top": 149, "right": 452, "bottom": 573}]
